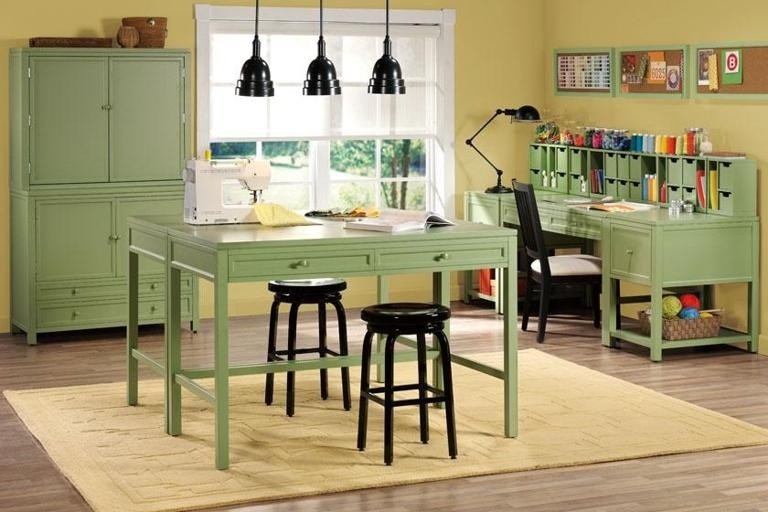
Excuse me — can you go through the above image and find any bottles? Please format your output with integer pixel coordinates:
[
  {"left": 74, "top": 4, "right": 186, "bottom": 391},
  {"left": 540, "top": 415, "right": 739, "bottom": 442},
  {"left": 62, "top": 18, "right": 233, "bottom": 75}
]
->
[
  {"left": 559, "top": 121, "right": 631, "bottom": 151},
  {"left": 631, "top": 127, "right": 708, "bottom": 155}
]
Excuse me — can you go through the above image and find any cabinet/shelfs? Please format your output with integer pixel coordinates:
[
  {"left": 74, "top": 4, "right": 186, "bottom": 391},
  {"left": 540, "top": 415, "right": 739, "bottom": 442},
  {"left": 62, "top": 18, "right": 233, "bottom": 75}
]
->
[
  {"left": 8, "top": 46, "right": 200, "bottom": 346},
  {"left": 464, "top": 143, "right": 761, "bottom": 361}
]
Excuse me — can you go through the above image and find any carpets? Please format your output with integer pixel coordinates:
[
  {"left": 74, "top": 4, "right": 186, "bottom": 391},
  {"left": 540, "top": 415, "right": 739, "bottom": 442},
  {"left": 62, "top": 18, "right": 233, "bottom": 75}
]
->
[{"left": 2, "top": 346, "right": 768, "bottom": 510}]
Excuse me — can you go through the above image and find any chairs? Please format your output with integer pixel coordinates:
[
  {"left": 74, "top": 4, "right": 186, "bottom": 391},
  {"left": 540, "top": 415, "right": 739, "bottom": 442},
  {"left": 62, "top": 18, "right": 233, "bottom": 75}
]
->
[{"left": 511, "top": 178, "right": 622, "bottom": 344}]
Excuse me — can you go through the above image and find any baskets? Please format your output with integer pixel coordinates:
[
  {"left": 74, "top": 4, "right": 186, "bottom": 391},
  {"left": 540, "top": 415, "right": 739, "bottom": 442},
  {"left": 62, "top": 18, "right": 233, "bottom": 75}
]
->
[
  {"left": 638, "top": 311, "right": 720, "bottom": 341},
  {"left": 123, "top": 16, "right": 168, "bottom": 48}
]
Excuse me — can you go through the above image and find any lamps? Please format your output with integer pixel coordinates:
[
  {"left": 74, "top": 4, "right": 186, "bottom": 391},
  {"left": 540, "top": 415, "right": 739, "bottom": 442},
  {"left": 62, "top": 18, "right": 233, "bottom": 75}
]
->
[
  {"left": 365, "top": 0, "right": 407, "bottom": 94},
  {"left": 235, "top": 0, "right": 274, "bottom": 97},
  {"left": 301, "top": 0, "right": 342, "bottom": 96},
  {"left": 465, "top": 105, "right": 540, "bottom": 193}
]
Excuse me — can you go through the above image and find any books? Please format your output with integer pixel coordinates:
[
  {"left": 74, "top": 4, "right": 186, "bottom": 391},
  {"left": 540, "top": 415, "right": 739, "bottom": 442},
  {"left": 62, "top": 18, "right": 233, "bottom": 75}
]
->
[
  {"left": 567, "top": 202, "right": 658, "bottom": 212},
  {"left": 344, "top": 208, "right": 455, "bottom": 235}
]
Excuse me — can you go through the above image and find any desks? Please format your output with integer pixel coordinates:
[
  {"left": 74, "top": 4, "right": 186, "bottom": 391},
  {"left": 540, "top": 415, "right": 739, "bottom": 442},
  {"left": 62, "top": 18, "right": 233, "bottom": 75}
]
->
[
  {"left": 127, "top": 216, "right": 439, "bottom": 431},
  {"left": 168, "top": 223, "right": 519, "bottom": 469}
]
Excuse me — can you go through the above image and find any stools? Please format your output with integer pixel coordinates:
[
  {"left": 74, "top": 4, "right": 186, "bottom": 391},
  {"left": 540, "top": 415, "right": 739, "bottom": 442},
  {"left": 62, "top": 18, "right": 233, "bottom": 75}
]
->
[
  {"left": 357, "top": 302, "right": 458, "bottom": 465},
  {"left": 265, "top": 278, "right": 352, "bottom": 417}
]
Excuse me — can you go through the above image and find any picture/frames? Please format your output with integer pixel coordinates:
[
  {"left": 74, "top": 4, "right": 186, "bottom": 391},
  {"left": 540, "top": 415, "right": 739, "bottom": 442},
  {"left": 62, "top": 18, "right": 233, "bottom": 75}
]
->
[
  {"left": 616, "top": 45, "right": 689, "bottom": 101},
  {"left": 551, "top": 47, "right": 613, "bottom": 97},
  {"left": 690, "top": 42, "right": 768, "bottom": 101}
]
